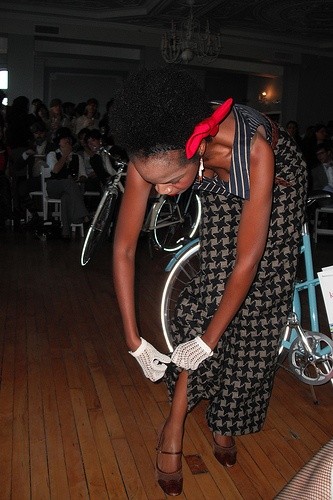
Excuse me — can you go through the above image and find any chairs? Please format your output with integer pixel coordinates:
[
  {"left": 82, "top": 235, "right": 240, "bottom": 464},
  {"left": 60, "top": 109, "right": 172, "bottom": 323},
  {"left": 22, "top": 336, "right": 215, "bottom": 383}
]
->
[{"left": 10, "top": 150, "right": 333, "bottom": 243}]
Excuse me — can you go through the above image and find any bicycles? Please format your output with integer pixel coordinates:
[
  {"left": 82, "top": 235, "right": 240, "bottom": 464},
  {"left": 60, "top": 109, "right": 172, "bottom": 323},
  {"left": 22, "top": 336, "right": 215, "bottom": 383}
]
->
[
  {"left": 79, "top": 144, "right": 202, "bottom": 267},
  {"left": 159, "top": 190, "right": 333, "bottom": 404}
]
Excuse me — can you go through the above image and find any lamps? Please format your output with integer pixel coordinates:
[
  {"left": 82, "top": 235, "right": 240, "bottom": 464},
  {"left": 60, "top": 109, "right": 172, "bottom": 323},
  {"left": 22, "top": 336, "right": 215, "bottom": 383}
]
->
[{"left": 159, "top": 0, "right": 224, "bottom": 65}]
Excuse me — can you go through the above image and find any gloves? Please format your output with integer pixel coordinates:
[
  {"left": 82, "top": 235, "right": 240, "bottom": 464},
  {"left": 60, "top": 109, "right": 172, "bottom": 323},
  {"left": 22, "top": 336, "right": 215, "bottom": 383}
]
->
[
  {"left": 129, "top": 337, "right": 171, "bottom": 381},
  {"left": 170, "top": 336, "right": 213, "bottom": 371}
]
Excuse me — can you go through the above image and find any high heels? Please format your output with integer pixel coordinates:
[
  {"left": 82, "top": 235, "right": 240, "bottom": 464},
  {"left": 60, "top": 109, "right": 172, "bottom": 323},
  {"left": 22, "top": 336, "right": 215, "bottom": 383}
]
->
[
  {"left": 156, "top": 446, "right": 183, "bottom": 496},
  {"left": 211, "top": 430, "right": 238, "bottom": 468}
]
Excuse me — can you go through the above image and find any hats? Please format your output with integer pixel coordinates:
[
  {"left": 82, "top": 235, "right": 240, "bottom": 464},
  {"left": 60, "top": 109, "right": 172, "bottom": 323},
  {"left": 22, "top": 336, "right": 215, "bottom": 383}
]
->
[{"left": 52, "top": 126, "right": 76, "bottom": 144}]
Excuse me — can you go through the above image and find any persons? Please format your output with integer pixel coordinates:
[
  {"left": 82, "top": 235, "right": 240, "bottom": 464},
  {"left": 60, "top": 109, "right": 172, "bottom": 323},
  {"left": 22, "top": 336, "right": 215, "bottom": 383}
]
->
[
  {"left": 285, "top": 121, "right": 304, "bottom": 155},
  {"left": 308, "top": 143, "right": 333, "bottom": 238},
  {"left": 304, "top": 121, "right": 333, "bottom": 166},
  {"left": 108, "top": 67, "right": 307, "bottom": 497},
  {"left": 4, "top": 95, "right": 115, "bottom": 231},
  {"left": 86, "top": 129, "right": 129, "bottom": 243},
  {"left": 44, "top": 127, "right": 92, "bottom": 239}
]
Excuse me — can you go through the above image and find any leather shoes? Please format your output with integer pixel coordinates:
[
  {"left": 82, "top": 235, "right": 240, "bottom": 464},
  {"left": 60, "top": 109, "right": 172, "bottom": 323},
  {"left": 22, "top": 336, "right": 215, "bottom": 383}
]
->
[
  {"left": 61, "top": 227, "right": 71, "bottom": 239},
  {"left": 83, "top": 219, "right": 93, "bottom": 231}
]
